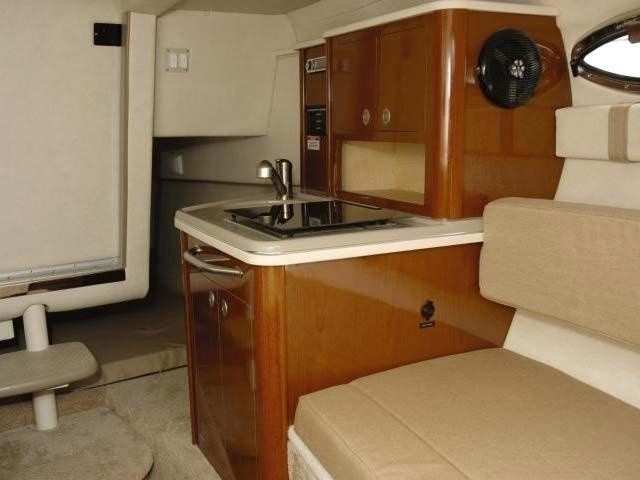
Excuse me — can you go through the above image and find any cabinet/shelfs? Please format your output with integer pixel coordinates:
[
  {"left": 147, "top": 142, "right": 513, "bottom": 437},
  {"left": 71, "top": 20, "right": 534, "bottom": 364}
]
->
[
  {"left": 324, "top": 6, "right": 568, "bottom": 147},
  {"left": 177, "top": 228, "right": 516, "bottom": 478}
]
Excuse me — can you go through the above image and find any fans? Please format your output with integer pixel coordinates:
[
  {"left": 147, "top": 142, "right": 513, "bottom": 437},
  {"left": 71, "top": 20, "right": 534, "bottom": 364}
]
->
[{"left": 480, "top": 28, "right": 541, "bottom": 108}]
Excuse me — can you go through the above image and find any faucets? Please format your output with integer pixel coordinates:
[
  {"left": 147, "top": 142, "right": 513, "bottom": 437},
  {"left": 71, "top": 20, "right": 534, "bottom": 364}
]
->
[
  {"left": 259, "top": 204, "right": 293, "bottom": 226},
  {"left": 256, "top": 158, "right": 292, "bottom": 200}
]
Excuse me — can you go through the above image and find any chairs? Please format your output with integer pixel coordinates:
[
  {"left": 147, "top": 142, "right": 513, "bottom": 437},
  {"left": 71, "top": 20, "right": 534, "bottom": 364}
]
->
[{"left": 287, "top": 198, "right": 640, "bottom": 480}]
